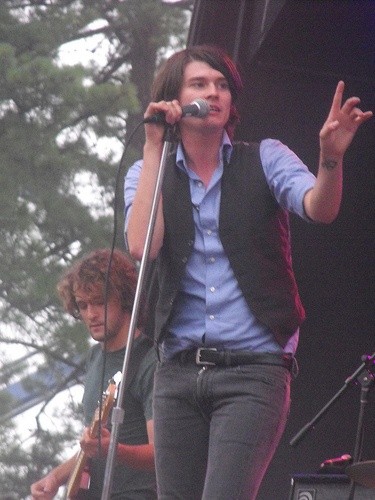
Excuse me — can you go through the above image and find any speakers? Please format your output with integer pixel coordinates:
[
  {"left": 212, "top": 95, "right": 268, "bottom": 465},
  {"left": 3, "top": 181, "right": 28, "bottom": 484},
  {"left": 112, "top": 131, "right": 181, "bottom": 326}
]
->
[{"left": 288, "top": 474, "right": 375, "bottom": 500}]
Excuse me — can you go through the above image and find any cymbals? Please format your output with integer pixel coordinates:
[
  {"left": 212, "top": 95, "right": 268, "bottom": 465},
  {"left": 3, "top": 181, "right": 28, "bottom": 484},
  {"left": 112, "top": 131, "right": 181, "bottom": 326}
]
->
[{"left": 346, "top": 460, "right": 375, "bottom": 488}]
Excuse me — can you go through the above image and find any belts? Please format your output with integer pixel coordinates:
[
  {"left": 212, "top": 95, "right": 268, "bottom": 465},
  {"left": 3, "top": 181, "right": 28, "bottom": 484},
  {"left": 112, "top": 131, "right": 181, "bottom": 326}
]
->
[{"left": 173, "top": 347, "right": 293, "bottom": 370}]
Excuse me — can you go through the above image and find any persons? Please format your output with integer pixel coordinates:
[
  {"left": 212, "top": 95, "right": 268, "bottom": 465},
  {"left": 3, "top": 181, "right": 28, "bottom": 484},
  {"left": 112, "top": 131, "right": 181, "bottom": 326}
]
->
[
  {"left": 31, "top": 250, "right": 160, "bottom": 500},
  {"left": 124, "top": 45, "right": 373, "bottom": 500}
]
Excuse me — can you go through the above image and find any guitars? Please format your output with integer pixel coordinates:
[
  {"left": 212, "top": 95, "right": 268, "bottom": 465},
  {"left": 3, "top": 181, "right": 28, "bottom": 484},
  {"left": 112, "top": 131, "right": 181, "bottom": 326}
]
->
[{"left": 53, "top": 378, "right": 117, "bottom": 500}]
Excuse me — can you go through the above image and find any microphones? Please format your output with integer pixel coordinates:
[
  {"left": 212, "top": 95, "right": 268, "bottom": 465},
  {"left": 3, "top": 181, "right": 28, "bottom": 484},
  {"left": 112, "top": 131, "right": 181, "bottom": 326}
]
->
[{"left": 144, "top": 99, "right": 210, "bottom": 124}]
